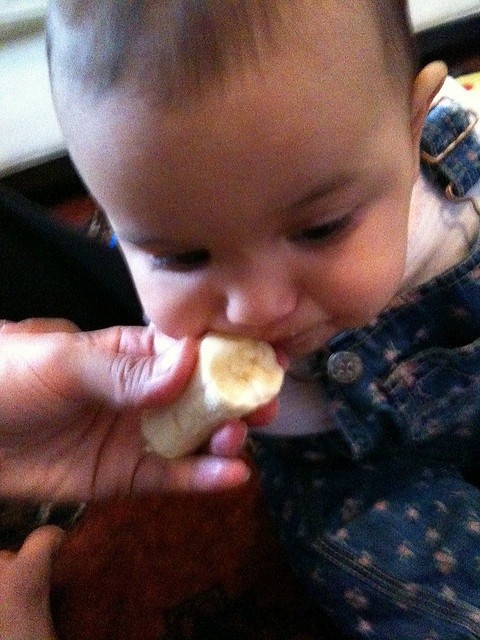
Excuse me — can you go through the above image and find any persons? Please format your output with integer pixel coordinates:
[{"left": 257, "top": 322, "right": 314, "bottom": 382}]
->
[
  {"left": 41, "top": 0, "right": 480, "bottom": 639},
  {"left": 2, "top": 314, "right": 290, "bottom": 639}
]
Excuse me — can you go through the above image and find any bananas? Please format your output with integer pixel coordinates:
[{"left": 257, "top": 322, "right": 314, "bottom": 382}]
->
[{"left": 140, "top": 331, "right": 286, "bottom": 461}]
